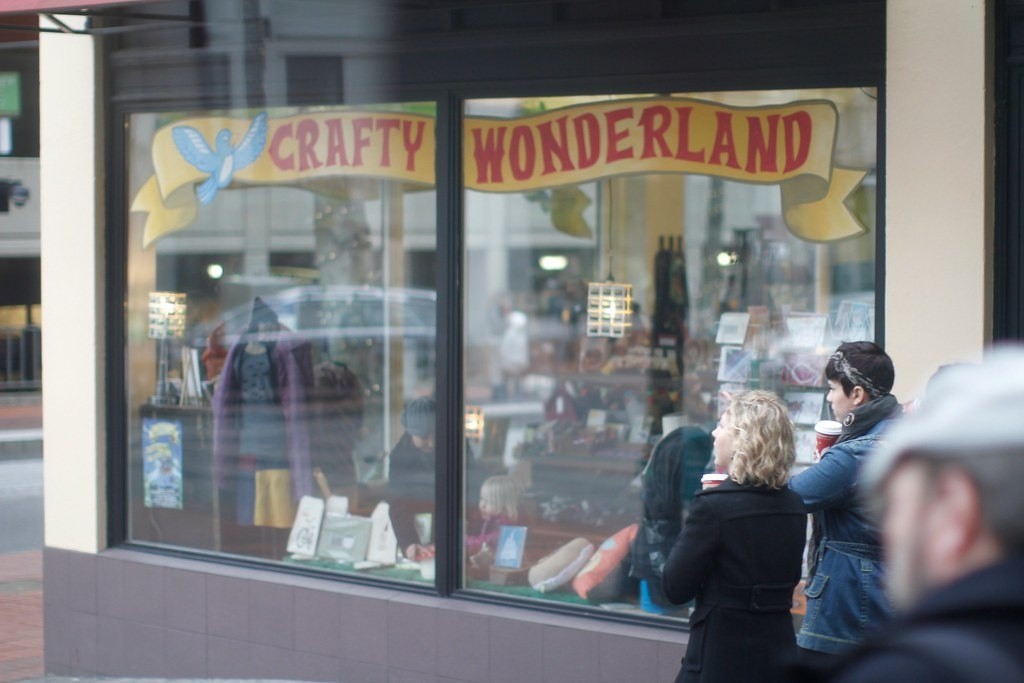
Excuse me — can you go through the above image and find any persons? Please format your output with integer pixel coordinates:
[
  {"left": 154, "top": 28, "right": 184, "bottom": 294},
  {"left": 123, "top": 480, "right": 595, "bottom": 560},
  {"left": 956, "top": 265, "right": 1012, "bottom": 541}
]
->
[
  {"left": 632, "top": 388, "right": 807, "bottom": 683},
  {"left": 215, "top": 295, "right": 318, "bottom": 561},
  {"left": 831, "top": 342, "right": 1023, "bottom": 683},
  {"left": 388, "top": 274, "right": 654, "bottom": 603},
  {"left": 787, "top": 343, "right": 901, "bottom": 683}
]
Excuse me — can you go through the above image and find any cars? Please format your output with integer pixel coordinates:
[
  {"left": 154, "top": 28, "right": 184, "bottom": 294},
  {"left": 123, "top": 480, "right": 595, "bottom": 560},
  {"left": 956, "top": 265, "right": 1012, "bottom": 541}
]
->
[{"left": 171, "top": 286, "right": 508, "bottom": 385}]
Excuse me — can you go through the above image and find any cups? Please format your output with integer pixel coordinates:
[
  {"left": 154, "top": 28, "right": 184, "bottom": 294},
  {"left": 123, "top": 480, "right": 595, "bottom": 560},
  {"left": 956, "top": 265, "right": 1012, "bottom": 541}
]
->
[
  {"left": 814, "top": 420, "right": 842, "bottom": 455},
  {"left": 415, "top": 513, "right": 432, "bottom": 544},
  {"left": 699, "top": 473, "right": 729, "bottom": 491}
]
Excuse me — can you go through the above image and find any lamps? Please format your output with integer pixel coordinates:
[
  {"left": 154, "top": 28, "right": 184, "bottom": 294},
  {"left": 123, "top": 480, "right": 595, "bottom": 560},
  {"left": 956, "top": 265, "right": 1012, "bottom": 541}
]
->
[
  {"left": 585, "top": 177, "right": 635, "bottom": 341},
  {"left": 147, "top": 292, "right": 188, "bottom": 404}
]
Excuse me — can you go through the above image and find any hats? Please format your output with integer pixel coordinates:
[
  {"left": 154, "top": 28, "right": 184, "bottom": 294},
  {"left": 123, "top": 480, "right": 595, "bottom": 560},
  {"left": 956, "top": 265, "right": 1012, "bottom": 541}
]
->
[
  {"left": 859, "top": 343, "right": 1024, "bottom": 483},
  {"left": 405, "top": 395, "right": 436, "bottom": 435}
]
